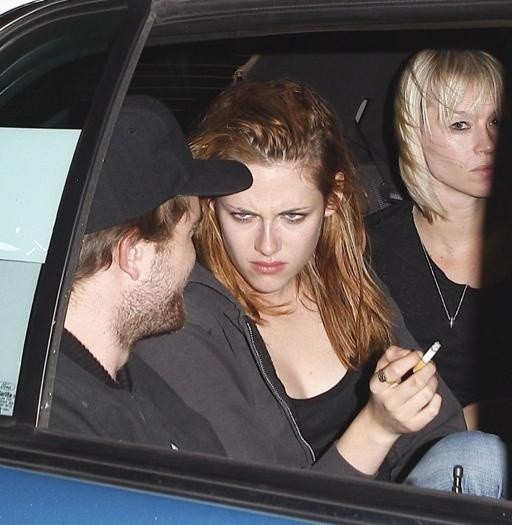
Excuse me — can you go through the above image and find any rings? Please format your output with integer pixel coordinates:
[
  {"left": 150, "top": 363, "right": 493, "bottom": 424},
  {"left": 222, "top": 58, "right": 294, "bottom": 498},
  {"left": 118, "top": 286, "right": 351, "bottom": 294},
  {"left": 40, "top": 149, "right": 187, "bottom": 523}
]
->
[{"left": 378, "top": 370, "right": 393, "bottom": 386}]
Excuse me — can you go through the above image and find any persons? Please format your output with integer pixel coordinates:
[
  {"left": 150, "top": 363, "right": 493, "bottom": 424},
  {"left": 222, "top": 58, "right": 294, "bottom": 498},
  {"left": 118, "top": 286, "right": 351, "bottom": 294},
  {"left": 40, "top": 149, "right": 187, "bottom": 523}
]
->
[
  {"left": 135, "top": 76, "right": 512, "bottom": 500},
  {"left": 360, "top": 47, "right": 512, "bottom": 433},
  {"left": 47, "top": 91, "right": 253, "bottom": 459}
]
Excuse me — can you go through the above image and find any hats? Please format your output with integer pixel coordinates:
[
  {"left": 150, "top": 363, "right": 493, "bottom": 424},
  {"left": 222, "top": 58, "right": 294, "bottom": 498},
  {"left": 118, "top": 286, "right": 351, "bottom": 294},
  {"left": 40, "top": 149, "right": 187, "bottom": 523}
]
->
[{"left": 86, "top": 93, "right": 253, "bottom": 233}]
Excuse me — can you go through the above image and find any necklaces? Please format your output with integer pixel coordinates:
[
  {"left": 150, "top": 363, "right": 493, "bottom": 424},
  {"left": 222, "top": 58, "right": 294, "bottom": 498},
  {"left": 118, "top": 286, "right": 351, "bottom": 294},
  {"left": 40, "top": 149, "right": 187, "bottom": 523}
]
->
[{"left": 413, "top": 203, "right": 476, "bottom": 331}]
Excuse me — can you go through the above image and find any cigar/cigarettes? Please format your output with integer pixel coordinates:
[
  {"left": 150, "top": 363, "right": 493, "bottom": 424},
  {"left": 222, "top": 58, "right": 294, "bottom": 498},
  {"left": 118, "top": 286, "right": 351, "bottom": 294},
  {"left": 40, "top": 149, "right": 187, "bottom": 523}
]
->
[{"left": 412, "top": 339, "right": 441, "bottom": 373}]
